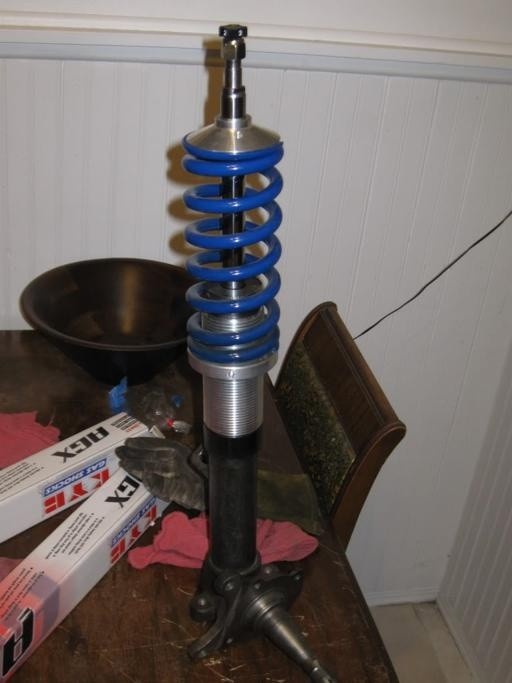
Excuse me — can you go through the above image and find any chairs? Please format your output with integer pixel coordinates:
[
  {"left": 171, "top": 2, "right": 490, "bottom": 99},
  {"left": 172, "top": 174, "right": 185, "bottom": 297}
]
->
[{"left": 274, "top": 302, "right": 405, "bottom": 552}]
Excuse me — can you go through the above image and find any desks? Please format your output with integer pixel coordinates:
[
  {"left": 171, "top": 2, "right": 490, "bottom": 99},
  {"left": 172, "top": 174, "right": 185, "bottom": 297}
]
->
[{"left": 0, "top": 329, "right": 400, "bottom": 683}]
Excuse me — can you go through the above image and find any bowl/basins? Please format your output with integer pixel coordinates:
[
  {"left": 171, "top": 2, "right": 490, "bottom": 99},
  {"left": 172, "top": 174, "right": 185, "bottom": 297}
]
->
[{"left": 17, "top": 256, "right": 207, "bottom": 378}]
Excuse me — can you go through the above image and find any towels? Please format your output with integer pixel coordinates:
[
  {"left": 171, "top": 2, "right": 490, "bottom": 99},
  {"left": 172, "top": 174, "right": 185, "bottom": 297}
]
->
[
  {"left": 0, "top": 411, "right": 60, "bottom": 469},
  {"left": 126, "top": 511, "right": 321, "bottom": 570}
]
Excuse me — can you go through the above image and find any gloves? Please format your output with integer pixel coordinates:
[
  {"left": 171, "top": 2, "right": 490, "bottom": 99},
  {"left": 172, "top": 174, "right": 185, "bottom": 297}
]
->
[{"left": 115, "top": 436, "right": 323, "bottom": 534}]
